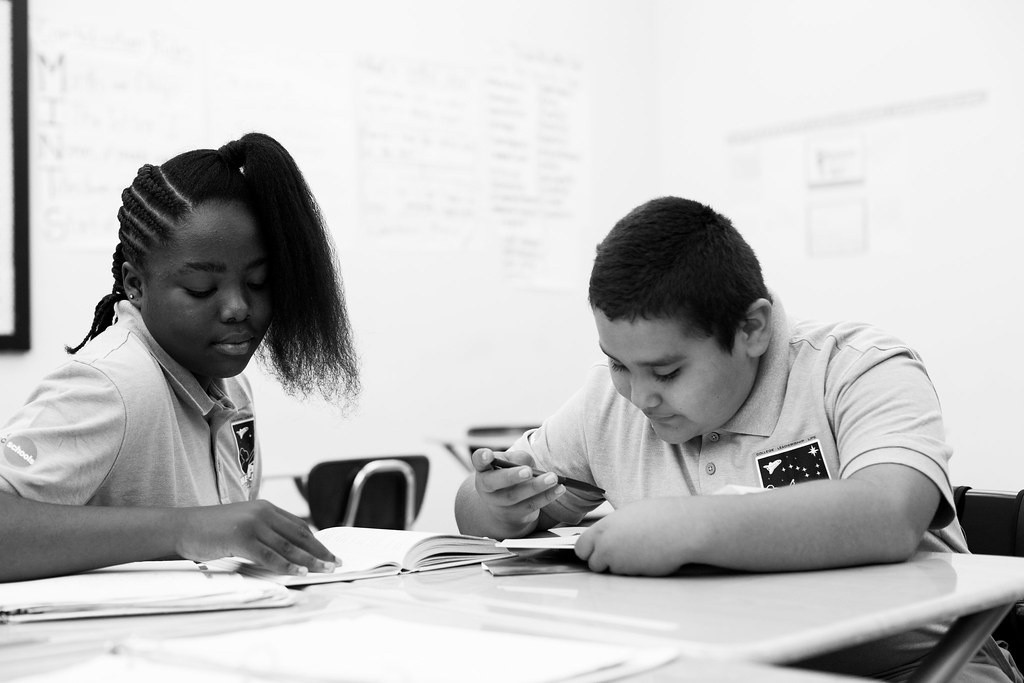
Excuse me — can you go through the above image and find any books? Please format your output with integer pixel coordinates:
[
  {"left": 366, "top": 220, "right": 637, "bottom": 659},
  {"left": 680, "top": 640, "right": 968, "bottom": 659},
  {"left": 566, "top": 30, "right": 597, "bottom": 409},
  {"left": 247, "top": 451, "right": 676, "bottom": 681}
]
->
[{"left": 0, "top": 523, "right": 589, "bottom": 625}]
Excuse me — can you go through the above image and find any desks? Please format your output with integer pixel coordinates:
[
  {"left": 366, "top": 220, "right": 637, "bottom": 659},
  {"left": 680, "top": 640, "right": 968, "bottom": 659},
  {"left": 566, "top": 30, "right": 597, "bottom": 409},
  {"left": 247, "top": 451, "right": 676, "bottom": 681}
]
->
[{"left": 0, "top": 544, "right": 1024, "bottom": 683}]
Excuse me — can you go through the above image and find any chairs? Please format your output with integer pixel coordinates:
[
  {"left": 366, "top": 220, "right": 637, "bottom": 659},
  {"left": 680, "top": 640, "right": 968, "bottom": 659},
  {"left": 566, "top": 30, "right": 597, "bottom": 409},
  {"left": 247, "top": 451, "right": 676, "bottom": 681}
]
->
[{"left": 305, "top": 453, "right": 430, "bottom": 532}]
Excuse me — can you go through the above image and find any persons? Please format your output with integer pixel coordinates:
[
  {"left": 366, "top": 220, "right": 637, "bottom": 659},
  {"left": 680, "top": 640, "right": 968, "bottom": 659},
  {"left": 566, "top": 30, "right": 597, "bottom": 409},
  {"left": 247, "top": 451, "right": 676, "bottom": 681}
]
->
[
  {"left": 452, "top": 196, "right": 1024, "bottom": 683},
  {"left": 0, "top": 133, "right": 366, "bottom": 573}
]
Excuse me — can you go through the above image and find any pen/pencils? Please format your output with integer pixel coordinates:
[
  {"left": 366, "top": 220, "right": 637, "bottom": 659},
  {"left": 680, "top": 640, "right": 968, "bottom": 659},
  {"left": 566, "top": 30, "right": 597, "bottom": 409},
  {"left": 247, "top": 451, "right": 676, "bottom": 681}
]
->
[{"left": 490, "top": 457, "right": 607, "bottom": 494}]
